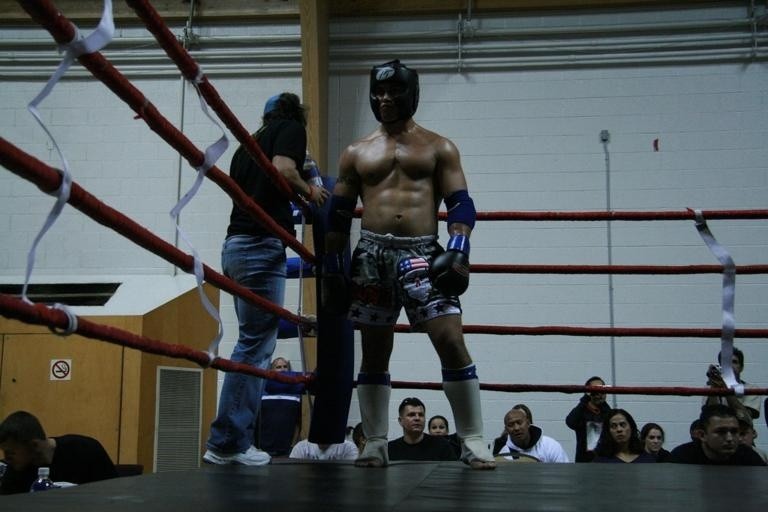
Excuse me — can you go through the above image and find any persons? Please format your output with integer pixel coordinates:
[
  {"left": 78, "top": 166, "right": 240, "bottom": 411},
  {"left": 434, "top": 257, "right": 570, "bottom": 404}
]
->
[
  {"left": 493, "top": 404, "right": 568, "bottom": 464},
  {"left": 566, "top": 347, "right": 768, "bottom": 466},
  {"left": 322, "top": 58, "right": 497, "bottom": 471},
  {"left": 0, "top": 409, "right": 119, "bottom": 494},
  {"left": 200, "top": 93, "right": 331, "bottom": 465},
  {"left": 264, "top": 357, "right": 463, "bottom": 462}
]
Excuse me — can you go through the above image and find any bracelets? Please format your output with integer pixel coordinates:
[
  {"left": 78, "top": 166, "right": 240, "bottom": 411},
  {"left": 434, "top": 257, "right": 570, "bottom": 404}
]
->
[{"left": 307, "top": 183, "right": 317, "bottom": 200}]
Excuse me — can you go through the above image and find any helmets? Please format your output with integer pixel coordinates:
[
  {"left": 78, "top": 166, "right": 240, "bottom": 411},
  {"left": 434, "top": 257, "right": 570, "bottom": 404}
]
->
[{"left": 369, "top": 58, "right": 419, "bottom": 125}]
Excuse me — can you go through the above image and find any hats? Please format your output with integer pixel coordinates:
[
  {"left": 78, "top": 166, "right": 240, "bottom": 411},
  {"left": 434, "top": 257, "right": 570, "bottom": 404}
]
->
[{"left": 262, "top": 93, "right": 311, "bottom": 118}]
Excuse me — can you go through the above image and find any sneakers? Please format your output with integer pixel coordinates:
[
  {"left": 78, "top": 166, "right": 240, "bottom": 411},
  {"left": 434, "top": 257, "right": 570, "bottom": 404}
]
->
[{"left": 202, "top": 441, "right": 271, "bottom": 466}]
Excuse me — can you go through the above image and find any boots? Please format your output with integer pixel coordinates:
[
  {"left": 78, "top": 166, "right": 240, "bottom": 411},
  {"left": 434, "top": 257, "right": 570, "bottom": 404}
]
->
[
  {"left": 439, "top": 364, "right": 496, "bottom": 469},
  {"left": 354, "top": 373, "right": 391, "bottom": 468}
]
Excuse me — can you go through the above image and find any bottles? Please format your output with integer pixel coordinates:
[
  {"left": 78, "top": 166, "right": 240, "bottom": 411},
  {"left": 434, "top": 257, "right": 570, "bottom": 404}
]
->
[
  {"left": 28, "top": 465, "right": 56, "bottom": 493},
  {"left": 301, "top": 150, "right": 326, "bottom": 195}
]
